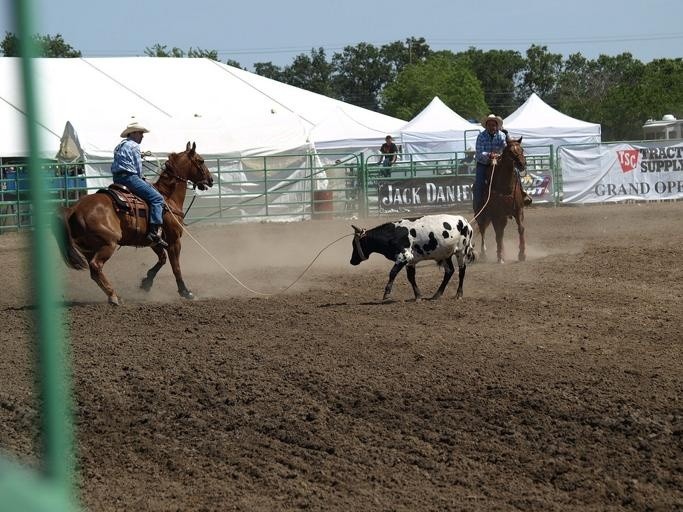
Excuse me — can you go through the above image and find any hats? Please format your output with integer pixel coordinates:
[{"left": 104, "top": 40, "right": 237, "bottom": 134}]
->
[
  {"left": 121, "top": 123, "right": 149, "bottom": 138},
  {"left": 481, "top": 114, "right": 503, "bottom": 129}
]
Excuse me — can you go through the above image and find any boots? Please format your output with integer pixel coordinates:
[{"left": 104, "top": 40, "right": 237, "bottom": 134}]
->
[{"left": 147, "top": 224, "right": 169, "bottom": 246}]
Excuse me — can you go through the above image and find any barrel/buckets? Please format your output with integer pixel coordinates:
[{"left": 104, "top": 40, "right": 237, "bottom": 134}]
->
[{"left": 312, "top": 191, "right": 333, "bottom": 220}]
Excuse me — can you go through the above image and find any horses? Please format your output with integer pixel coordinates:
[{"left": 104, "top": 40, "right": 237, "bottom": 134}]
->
[
  {"left": 50, "top": 141, "right": 213, "bottom": 305},
  {"left": 474, "top": 134, "right": 527, "bottom": 264}
]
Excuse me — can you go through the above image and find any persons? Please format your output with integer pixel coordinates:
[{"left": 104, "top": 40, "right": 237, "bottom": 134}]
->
[
  {"left": 473, "top": 114, "right": 507, "bottom": 210},
  {"left": 377, "top": 135, "right": 397, "bottom": 178},
  {"left": 111, "top": 122, "right": 169, "bottom": 248}
]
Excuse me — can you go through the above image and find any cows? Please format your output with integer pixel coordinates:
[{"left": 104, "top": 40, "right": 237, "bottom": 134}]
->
[{"left": 350, "top": 214, "right": 476, "bottom": 303}]
